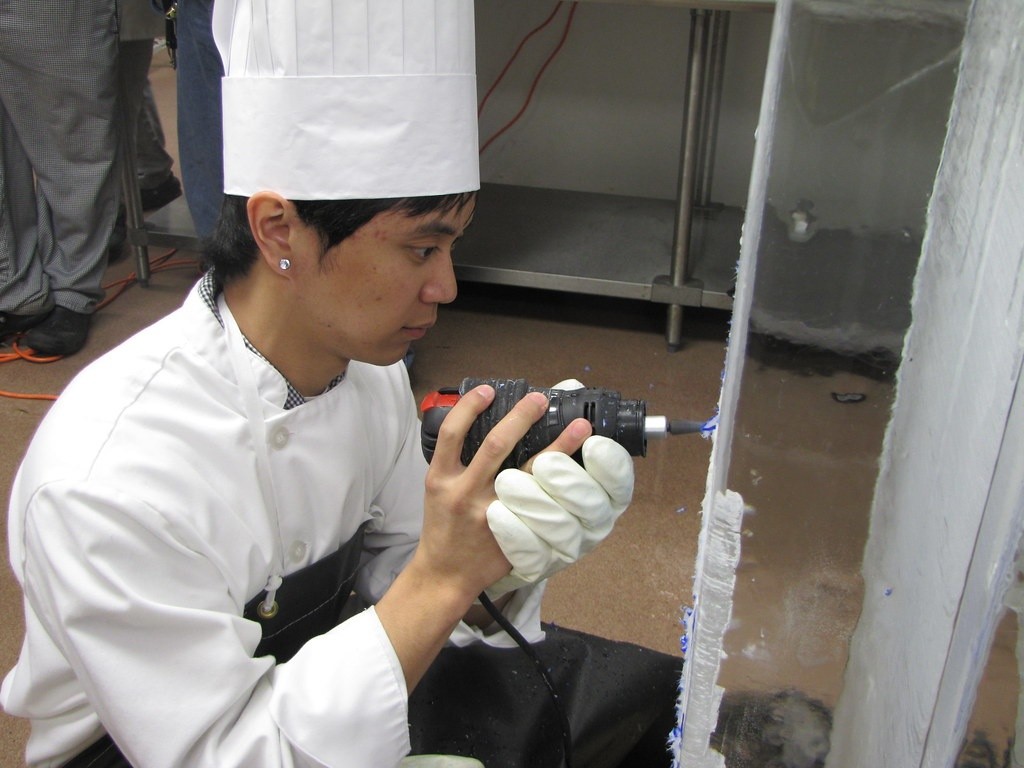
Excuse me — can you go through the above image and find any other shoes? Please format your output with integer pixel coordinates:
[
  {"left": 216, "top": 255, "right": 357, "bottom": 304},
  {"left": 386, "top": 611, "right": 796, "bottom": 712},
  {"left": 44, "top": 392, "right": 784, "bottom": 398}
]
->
[
  {"left": 109, "top": 205, "right": 127, "bottom": 260},
  {"left": 140, "top": 170, "right": 182, "bottom": 209},
  {"left": 25, "top": 306, "right": 90, "bottom": 354},
  {"left": 0, "top": 311, "right": 44, "bottom": 343}
]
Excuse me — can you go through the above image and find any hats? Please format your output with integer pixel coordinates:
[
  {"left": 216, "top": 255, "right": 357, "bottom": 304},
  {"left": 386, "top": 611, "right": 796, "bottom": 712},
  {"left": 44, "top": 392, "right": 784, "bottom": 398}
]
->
[{"left": 212, "top": 0, "right": 480, "bottom": 200}]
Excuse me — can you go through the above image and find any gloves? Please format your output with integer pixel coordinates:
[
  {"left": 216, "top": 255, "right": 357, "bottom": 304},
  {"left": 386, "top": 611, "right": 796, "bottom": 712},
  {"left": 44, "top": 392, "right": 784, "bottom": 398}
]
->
[{"left": 469, "top": 378, "right": 634, "bottom": 607}]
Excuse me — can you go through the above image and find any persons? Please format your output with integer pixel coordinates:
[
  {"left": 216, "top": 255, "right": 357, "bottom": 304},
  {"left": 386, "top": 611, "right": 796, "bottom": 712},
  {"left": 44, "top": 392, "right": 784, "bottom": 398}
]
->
[
  {"left": 0, "top": 1, "right": 121, "bottom": 359},
  {"left": 178, "top": 0, "right": 223, "bottom": 238},
  {"left": 115, "top": 1, "right": 181, "bottom": 211},
  {"left": 0, "top": 0, "right": 635, "bottom": 768}
]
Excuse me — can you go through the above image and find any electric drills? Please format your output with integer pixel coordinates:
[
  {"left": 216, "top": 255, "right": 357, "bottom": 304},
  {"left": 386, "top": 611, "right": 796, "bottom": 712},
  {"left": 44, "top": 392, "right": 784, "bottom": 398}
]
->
[{"left": 419, "top": 376, "right": 713, "bottom": 479}]
